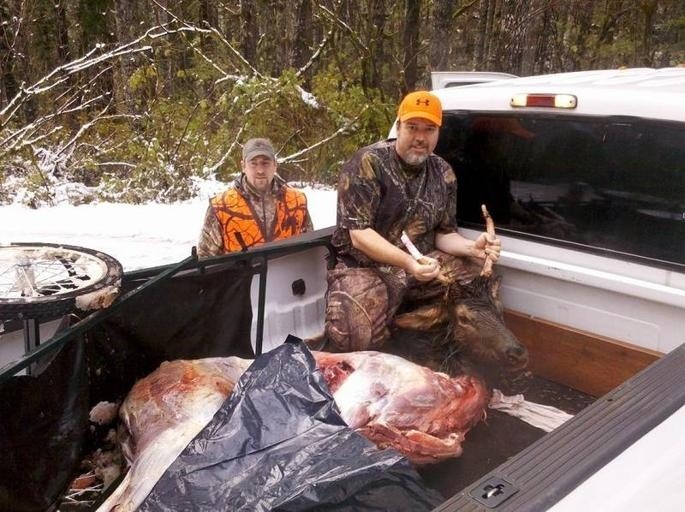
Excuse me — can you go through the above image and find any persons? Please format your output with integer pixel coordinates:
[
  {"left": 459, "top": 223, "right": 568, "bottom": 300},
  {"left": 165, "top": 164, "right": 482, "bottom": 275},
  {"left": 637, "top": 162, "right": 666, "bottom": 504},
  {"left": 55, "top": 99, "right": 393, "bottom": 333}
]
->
[
  {"left": 324, "top": 91, "right": 500, "bottom": 351},
  {"left": 198, "top": 138, "right": 314, "bottom": 258},
  {"left": 444, "top": 113, "right": 534, "bottom": 229}
]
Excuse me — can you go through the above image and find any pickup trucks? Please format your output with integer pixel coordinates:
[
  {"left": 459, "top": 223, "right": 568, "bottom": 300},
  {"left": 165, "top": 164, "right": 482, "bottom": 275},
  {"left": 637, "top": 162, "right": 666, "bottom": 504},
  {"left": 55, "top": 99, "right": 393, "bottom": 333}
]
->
[{"left": 2, "top": 57, "right": 685, "bottom": 511}]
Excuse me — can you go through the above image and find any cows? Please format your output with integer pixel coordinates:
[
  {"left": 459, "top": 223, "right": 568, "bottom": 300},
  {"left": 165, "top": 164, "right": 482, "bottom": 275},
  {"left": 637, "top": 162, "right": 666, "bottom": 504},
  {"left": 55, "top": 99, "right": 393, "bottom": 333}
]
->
[{"left": 89, "top": 203, "right": 529, "bottom": 512}]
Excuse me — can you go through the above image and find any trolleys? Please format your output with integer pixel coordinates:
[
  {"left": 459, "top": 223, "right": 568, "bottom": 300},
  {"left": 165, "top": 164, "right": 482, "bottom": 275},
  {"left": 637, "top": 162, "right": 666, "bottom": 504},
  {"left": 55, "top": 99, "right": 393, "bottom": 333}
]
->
[{"left": 0, "top": 237, "right": 338, "bottom": 512}]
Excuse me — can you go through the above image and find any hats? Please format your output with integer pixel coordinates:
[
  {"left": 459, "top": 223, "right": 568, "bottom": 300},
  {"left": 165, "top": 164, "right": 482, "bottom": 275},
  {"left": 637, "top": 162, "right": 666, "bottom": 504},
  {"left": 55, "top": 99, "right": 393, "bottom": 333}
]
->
[
  {"left": 398, "top": 91, "right": 444, "bottom": 127},
  {"left": 241, "top": 138, "right": 275, "bottom": 163}
]
